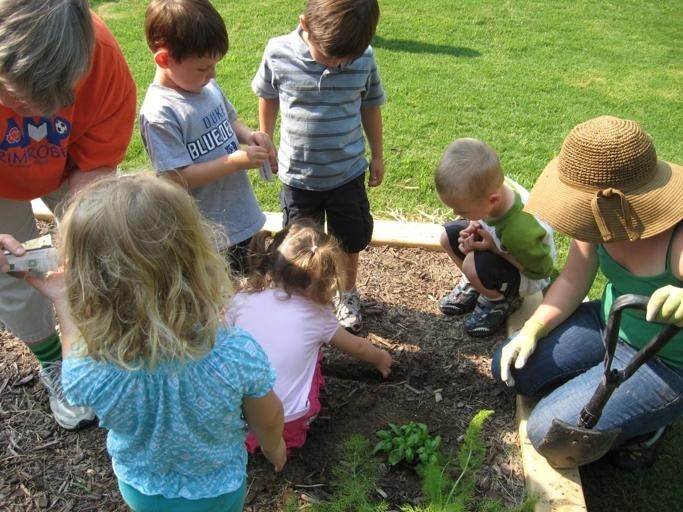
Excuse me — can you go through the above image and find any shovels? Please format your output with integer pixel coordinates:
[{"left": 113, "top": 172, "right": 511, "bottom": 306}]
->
[{"left": 534, "top": 293, "right": 683, "bottom": 469}]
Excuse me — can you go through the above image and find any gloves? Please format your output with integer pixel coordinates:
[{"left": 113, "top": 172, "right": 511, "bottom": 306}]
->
[
  {"left": 499, "top": 316, "right": 549, "bottom": 387},
  {"left": 644, "top": 284, "right": 683, "bottom": 325}
]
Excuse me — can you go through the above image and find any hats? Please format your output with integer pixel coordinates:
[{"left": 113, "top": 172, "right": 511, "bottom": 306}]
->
[{"left": 522, "top": 115, "right": 683, "bottom": 244}]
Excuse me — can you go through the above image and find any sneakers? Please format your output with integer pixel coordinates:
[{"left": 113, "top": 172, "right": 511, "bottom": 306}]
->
[
  {"left": 618, "top": 427, "right": 665, "bottom": 469},
  {"left": 332, "top": 287, "right": 363, "bottom": 333},
  {"left": 465, "top": 293, "right": 524, "bottom": 338},
  {"left": 39, "top": 359, "right": 96, "bottom": 431},
  {"left": 439, "top": 280, "right": 479, "bottom": 317}
]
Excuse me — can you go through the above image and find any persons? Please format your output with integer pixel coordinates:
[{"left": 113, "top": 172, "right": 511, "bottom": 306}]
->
[
  {"left": 250, "top": 0, "right": 385, "bottom": 335},
  {"left": 435, "top": 137, "right": 556, "bottom": 336},
  {"left": 0, "top": 0, "right": 137, "bottom": 431},
  {"left": 217, "top": 220, "right": 393, "bottom": 451},
  {"left": 491, "top": 115, "right": 683, "bottom": 471},
  {"left": 23, "top": 172, "right": 288, "bottom": 511},
  {"left": 137, "top": 0, "right": 272, "bottom": 277}
]
